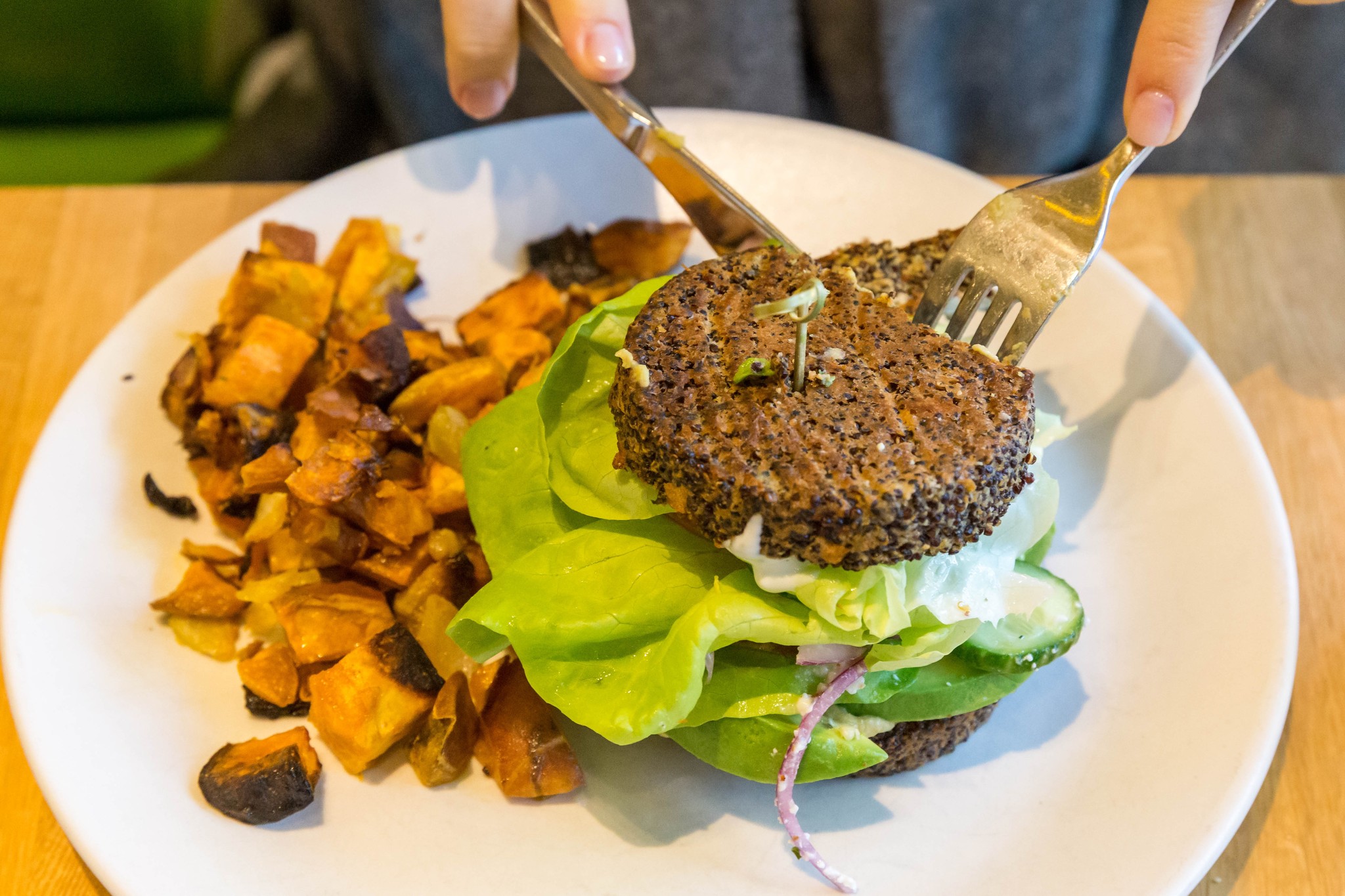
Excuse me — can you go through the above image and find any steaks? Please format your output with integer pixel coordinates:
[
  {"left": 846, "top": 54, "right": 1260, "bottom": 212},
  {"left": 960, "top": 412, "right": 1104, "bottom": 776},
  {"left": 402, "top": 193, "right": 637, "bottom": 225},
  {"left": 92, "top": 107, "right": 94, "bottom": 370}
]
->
[{"left": 608, "top": 245, "right": 1038, "bottom": 568}]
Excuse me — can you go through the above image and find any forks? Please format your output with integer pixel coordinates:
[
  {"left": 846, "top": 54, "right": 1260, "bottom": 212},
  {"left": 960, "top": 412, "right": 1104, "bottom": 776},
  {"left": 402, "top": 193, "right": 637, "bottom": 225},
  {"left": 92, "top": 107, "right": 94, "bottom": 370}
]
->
[{"left": 918, "top": 6, "right": 1294, "bottom": 366}]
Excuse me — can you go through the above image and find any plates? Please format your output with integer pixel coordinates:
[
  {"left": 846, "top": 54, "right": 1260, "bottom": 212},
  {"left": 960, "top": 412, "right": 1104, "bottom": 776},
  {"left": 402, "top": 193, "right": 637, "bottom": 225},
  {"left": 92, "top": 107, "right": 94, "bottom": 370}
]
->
[{"left": 0, "top": 105, "right": 1304, "bottom": 896}]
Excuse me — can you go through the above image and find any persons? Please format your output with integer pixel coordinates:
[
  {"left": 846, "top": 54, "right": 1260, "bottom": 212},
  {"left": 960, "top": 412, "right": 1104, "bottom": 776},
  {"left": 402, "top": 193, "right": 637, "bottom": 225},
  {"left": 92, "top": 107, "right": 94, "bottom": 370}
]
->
[{"left": 284, "top": 0, "right": 1344, "bottom": 177}]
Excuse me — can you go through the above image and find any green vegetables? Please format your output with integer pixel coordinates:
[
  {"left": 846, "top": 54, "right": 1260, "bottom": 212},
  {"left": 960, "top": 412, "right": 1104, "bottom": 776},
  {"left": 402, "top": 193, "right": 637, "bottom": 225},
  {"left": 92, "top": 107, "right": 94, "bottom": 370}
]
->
[{"left": 446, "top": 275, "right": 1089, "bottom": 785}]
